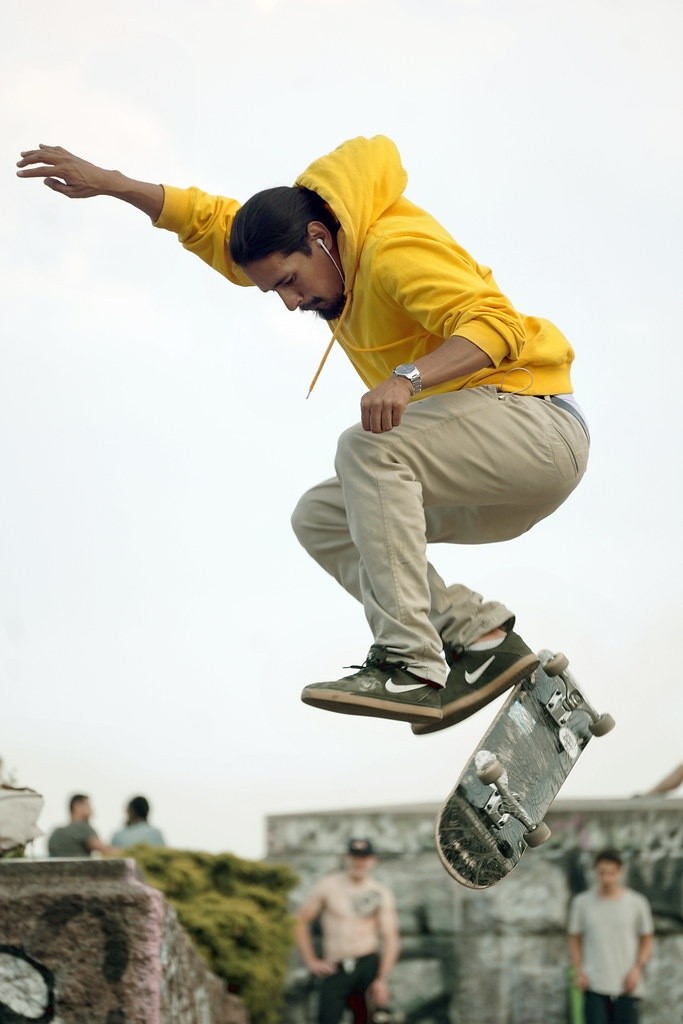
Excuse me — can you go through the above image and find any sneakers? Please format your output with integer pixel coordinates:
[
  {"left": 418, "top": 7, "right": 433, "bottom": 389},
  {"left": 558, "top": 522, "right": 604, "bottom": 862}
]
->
[
  {"left": 411, "top": 628, "right": 541, "bottom": 734},
  {"left": 301, "top": 644, "right": 444, "bottom": 725}
]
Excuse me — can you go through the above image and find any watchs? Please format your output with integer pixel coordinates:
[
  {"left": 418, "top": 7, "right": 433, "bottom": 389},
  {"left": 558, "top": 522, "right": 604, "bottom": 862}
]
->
[{"left": 393, "top": 363, "right": 422, "bottom": 394}]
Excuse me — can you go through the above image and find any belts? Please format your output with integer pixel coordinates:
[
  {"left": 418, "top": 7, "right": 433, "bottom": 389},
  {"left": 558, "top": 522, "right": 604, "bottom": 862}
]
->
[{"left": 535, "top": 394, "right": 590, "bottom": 442}]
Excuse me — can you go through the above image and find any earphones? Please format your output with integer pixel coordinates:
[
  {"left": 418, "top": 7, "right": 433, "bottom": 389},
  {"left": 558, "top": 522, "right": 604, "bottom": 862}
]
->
[{"left": 316, "top": 238, "right": 329, "bottom": 254}]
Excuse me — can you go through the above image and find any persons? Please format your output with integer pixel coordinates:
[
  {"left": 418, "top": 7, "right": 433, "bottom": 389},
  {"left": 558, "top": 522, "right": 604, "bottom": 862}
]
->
[
  {"left": 16, "top": 137, "right": 588, "bottom": 736},
  {"left": 294, "top": 835, "right": 397, "bottom": 1024},
  {"left": 567, "top": 850, "right": 654, "bottom": 1024},
  {"left": 50, "top": 794, "right": 118, "bottom": 857},
  {"left": 110, "top": 797, "right": 165, "bottom": 850}
]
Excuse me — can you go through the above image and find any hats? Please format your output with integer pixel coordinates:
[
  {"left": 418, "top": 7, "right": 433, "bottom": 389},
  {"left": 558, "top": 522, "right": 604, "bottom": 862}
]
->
[
  {"left": 348, "top": 838, "right": 372, "bottom": 856},
  {"left": 596, "top": 848, "right": 623, "bottom": 864}
]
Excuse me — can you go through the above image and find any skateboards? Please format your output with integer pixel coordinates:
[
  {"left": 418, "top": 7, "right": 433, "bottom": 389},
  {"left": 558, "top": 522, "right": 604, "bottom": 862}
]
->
[{"left": 435, "top": 648, "right": 616, "bottom": 890}]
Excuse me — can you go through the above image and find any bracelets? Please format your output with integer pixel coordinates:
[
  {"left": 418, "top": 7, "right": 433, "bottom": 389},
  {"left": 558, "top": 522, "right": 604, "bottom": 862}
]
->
[
  {"left": 637, "top": 959, "right": 644, "bottom": 971},
  {"left": 375, "top": 970, "right": 389, "bottom": 984}
]
